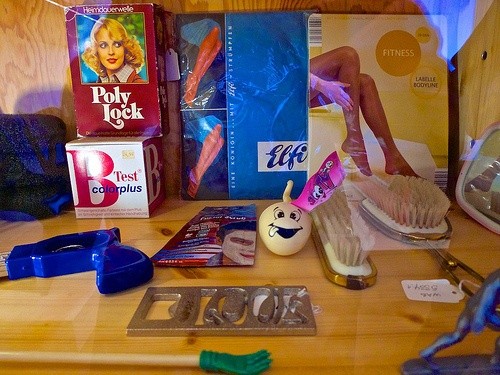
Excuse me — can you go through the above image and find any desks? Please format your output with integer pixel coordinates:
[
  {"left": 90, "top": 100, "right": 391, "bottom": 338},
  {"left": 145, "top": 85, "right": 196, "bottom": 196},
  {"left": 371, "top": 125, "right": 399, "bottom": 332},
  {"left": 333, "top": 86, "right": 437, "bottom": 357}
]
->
[{"left": 1, "top": 196, "right": 500, "bottom": 374}]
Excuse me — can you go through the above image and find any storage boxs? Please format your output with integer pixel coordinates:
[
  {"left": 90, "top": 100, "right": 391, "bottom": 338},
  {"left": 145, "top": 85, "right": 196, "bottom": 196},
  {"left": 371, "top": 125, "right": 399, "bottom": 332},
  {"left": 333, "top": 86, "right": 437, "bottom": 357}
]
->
[
  {"left": 64, "top": 137, "right": 167, "bottom": 219},
  {"left": 64, "top": 1, "right": 171, "bottom": 140}
]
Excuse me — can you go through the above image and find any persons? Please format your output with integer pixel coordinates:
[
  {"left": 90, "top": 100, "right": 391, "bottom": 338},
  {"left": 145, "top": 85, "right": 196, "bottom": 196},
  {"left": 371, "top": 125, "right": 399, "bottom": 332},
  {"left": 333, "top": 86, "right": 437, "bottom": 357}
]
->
[
  {"left": 80, "top": 17, "right": 147, "bottom": 84},
  {"left": 183, "top": 111, "right": 224, "bottom": 199},
  {"left": 209, "top": 220, "right": 257, "bottom": 266},
  {"left": 416, "top": 267, "right": 499, "bottom": 374},
  {"left": 179, "top": 18, "right": 228, "bottom": 112},
  {"left": 308, "top": 46, "right": 423, "bottom": 182}
]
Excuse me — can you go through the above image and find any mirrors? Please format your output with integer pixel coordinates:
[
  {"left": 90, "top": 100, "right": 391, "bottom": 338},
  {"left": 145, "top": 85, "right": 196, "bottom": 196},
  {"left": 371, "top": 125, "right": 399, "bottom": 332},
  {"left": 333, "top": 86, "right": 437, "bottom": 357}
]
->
[{"left": 456, "top": 121, "right": 499, "bottom": 235}]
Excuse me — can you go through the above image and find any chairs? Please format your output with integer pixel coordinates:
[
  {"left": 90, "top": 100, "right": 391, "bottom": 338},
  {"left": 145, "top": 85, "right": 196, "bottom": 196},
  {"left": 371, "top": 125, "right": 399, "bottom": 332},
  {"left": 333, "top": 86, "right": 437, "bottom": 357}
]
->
[{"left": 0, "top": 114, "right": 71, "bottom": 222}]
